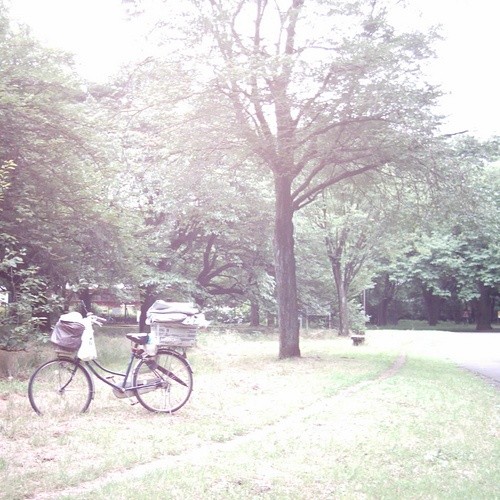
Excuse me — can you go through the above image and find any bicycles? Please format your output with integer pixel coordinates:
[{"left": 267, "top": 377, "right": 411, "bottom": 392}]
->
[{"left": 28, "top": 301, "right": 193, "bottom": 417}]
